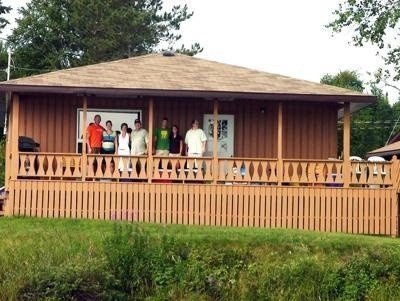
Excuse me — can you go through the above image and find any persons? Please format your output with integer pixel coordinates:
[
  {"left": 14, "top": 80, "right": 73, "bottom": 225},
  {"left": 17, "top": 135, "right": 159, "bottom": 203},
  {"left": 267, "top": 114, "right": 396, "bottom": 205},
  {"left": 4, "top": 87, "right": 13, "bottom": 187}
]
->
[
  {"left": 80, "top": 115, "right": 207, "bottom": 184},
  {"left": 207, "top": 120, "right": 227, "bottom": 140}
]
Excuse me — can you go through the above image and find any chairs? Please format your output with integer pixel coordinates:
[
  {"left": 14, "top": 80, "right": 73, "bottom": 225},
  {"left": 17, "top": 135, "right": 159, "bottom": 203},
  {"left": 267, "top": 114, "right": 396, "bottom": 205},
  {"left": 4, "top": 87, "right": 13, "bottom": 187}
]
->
[
  {"left": 19, "top": 134, "right": 41, "bottom": 169},
  {"left": 349, "top": 156, "right": 364, "bottom": 174},
  {"left": 366, "top": 156, "right": 386, "bottom": 175}
]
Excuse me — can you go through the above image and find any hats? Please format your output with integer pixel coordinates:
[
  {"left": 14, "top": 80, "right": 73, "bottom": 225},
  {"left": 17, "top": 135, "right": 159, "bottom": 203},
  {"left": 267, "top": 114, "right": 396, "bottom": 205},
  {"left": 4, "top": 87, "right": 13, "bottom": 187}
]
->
[{"left": 135, "top": 119, "right": 140, "bottom": 123}]
